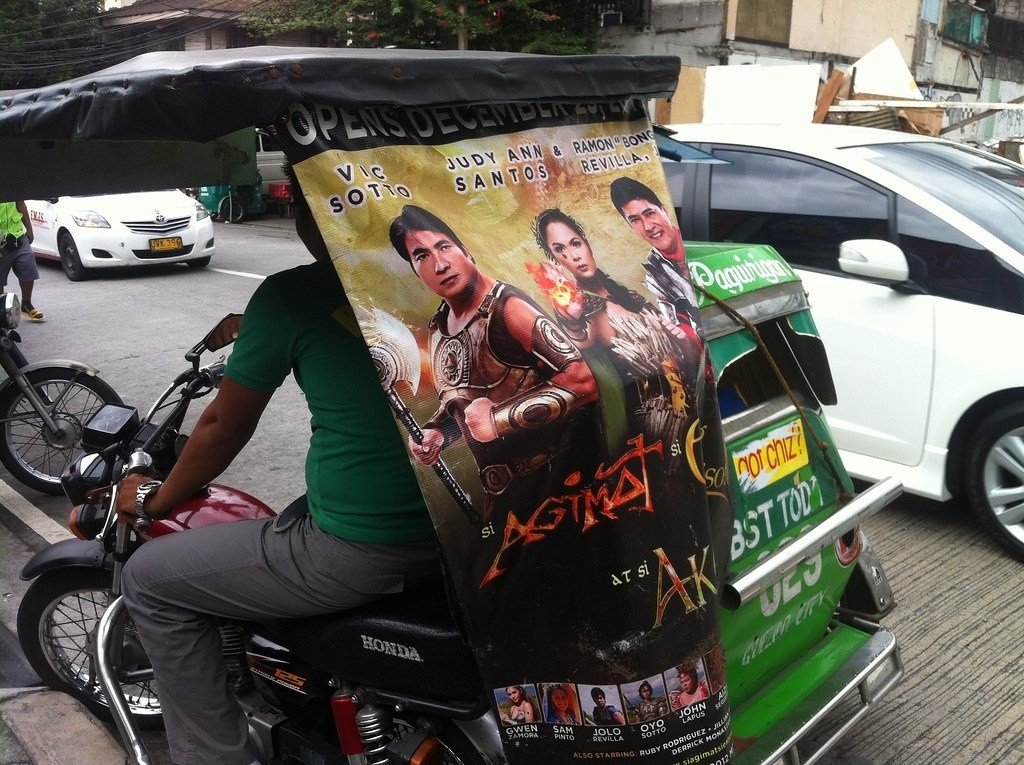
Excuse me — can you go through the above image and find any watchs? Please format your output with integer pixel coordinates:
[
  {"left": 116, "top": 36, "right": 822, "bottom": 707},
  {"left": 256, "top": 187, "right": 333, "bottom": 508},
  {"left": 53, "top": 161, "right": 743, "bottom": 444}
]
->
[{"left": 135, "top": 480, "right": 166, "bottom": 521}]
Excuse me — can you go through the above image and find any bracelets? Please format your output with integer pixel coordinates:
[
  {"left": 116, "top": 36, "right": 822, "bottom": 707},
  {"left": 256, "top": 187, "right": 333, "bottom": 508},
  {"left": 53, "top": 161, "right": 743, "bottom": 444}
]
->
[{"left": 553, "top": 303, "right": 590, "bottom": 344}]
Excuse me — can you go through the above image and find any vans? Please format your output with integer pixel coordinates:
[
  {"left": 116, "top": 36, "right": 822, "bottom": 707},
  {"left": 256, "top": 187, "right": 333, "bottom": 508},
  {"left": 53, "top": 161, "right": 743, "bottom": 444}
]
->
[{"left": 254, "top": 127, "right": 295, "bottom": 215}]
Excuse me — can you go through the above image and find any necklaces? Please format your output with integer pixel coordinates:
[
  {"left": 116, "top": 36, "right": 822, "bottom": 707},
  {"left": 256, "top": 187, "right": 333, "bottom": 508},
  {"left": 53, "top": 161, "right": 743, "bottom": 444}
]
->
[
  {"left": 594, "top": 291, "right": 618, "bottom": 305},
  {"left": 557, "top": 710, "right": 571, "bottom": 724}
]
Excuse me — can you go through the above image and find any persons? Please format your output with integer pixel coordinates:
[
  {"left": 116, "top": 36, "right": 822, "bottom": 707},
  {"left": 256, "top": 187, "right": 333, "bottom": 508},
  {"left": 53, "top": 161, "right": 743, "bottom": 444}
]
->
[
  {"left": 119, "top": 152, "right": 451, "bottom": 764},
  {"left": 633, "top": 680, "right": 667, "bottom": 725},
  {"left": 669, "top": 660, "right": 709, "bottom": 709},
  {"left": 387, "top": 204, "right": 615, "bottom": 673},
  {"left": 1, "top": 201, "right": 46, "bottom": 323},
  {"left": 499, "top": 686, "right": 536, "bottom": 728},
  {"left": 608, "top": 175, "right": 714, "bottom": 385},
  {"left": 590, "top": 686, "right": 625, "bottom": 726},
  {"left": 528, "top": 204, "right": 713, "bottom": 558},
  {"left": 543, "top": 684, "right": 581, "bottom": 725}
]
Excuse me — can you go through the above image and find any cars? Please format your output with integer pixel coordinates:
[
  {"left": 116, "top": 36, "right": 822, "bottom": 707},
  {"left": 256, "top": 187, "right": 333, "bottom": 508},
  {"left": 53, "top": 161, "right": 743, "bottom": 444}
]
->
[
  {"left": 649, "top": 120, "right": 1024, "bottom": 566},
  {"left": 25, "top": 188, "right": 217, "bottom": 283}
]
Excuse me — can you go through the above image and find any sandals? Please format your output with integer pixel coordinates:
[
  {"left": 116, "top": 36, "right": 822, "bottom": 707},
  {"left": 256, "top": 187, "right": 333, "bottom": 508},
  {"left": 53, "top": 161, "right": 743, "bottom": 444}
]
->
[{"left": 22, "top": 304, "right": 45, "bottom": 322}]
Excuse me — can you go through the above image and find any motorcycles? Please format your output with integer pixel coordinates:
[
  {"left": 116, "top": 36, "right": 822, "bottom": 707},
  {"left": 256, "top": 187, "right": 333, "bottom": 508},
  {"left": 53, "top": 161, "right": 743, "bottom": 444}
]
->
[
  {"left": 0, "top": 46, "right": 913, "bottom": 765},
  {"left": 0, "top": 291, "right": 124, "bottom": 495},
  {"left": 199, "top": 169, "right": 262, "bottom": 225}
]
